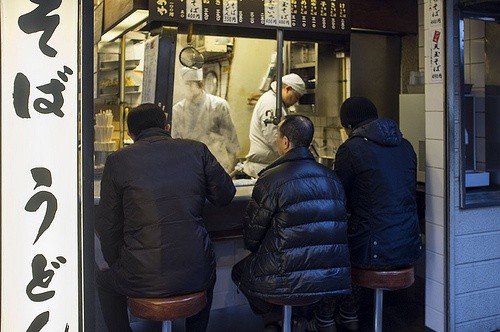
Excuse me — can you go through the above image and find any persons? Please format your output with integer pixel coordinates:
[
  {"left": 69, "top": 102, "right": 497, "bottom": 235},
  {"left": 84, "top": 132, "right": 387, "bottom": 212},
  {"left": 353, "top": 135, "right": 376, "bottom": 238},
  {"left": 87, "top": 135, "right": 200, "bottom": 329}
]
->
[
  {"left": 301, "top": 96, "right": 423, "bottom": 332},
  {"left": 99, "top": 102, "right": 236, "bottom": 332},
  {"left": 244, "top": 73, "right": 308, "bottom": 179},
  {"left": 172, "top": 65, "right": 237, "bottom": 178},
  {"left": 231, "top": 115, "right": 352, "bottom": 332}
]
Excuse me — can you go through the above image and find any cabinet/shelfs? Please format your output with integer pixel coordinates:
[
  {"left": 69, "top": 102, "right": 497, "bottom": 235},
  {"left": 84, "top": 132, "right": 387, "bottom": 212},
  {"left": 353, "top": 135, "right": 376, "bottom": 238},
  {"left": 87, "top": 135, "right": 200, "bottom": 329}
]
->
[{"left": 287, "top": 41, "right": 338, "bottom": 117}]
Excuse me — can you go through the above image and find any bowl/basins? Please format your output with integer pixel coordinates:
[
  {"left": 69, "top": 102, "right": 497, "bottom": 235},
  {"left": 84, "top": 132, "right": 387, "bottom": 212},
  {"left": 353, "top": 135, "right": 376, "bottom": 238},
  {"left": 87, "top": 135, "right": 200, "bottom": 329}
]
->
[{"left": 316, "top": 155, "right": 334, "bottom": 168}]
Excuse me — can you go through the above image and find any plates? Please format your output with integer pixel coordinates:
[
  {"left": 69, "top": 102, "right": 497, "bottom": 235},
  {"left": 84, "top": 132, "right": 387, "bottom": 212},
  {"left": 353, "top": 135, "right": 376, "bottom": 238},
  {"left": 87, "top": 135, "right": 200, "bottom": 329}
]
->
[{"left": 294, "top": 105, "right": 311, "bottom": 113}]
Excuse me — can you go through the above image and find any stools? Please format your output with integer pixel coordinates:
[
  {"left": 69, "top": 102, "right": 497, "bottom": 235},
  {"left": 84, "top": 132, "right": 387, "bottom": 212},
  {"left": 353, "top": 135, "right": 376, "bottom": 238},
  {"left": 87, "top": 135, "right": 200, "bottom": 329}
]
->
[
  {"left": 356, "top": 268, "right": 415, "bottom": 332},
  {"left": 264, "top": 297, "right": 320, "bottom": 332},
  {"left": 127, "top": 290, "right": 207, "bottom": 332}
]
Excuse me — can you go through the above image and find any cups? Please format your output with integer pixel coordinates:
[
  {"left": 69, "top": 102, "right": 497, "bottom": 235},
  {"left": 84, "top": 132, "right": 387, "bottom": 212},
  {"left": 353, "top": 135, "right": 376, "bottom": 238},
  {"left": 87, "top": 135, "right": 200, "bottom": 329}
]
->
[{"left": 94, "top": 150, "right": 110, "bottom": 166}]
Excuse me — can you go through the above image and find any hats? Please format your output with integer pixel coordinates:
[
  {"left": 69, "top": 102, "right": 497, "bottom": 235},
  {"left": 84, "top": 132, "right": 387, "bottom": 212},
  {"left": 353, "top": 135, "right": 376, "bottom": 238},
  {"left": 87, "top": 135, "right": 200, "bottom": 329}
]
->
[
  {"left": 282, "top": 72, "right": 306, "bottom": 96},
  {"left": 340, "top": 96, "right": 378, "bottom": 128},
  {"left": 182, "top": 66, "right": 203, "bottom": 81}
]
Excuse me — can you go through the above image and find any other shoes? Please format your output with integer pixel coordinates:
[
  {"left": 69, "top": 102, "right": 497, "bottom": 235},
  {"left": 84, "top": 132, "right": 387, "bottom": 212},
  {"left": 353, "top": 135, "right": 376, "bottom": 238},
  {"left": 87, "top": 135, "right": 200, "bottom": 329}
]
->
[
  {"left": 305, "top": 306, "right": 337, "bottom": 332},
  {"left": 335, "top": 303, "right": 361, "bottom": 332},
  {"left": 385, "top": 306, "right": 411, "bottom": 332},
  {"left": 401, "top": 302, "right": 426, "bottom": 332}
]
